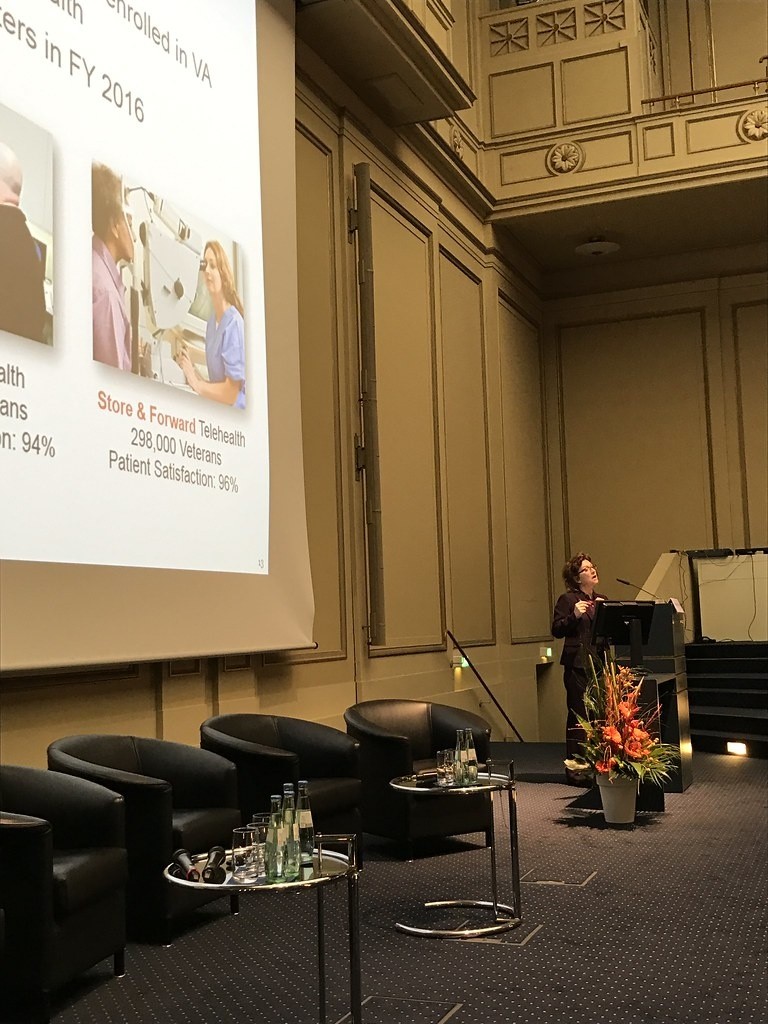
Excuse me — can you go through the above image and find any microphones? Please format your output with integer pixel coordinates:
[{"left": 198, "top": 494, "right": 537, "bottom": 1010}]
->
[
  {"left": 202, "top": 846, "right": 225, "bottom": 880},
  {"left": 174, "top": 849, "right": 200, "bottom": 881},
  {"left": 617, "top": 578, "right": 661, "bottom": 601}
]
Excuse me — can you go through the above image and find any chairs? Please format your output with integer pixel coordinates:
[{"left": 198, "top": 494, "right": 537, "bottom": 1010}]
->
[
  {"left": 48, "top": 733, "right": 243, "bottom": 949},
  {"left": 347, "top": 698, "right": 494, "bottom": 861},
  {"left": 0, "top": 764, "right": 130, "bottom": 1024},
  {"left": 200, "top": 712, "right": 374, "bottom": 896}
]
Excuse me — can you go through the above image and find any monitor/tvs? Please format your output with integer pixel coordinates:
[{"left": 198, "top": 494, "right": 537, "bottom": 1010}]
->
[{"left": 590, "top": 600, "right": 655, "bottom": 667}]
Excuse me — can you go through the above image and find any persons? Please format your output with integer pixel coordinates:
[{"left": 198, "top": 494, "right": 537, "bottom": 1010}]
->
[
  {"left": 551, "top": 554, "right": 609, "bottom": 782},
  {"left": 92, "top": 163, "right": 137, "bottom": 370},
  {"left": 177, "top": 241, "right": 245, "bottom": 408},
  {"left": 0, "top": 141, "right": 45, "bottom": 338}
]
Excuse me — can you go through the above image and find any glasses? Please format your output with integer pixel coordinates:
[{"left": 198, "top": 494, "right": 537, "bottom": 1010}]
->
[{"left": 577, "top": 564, "right": 598, "bottom": 575}]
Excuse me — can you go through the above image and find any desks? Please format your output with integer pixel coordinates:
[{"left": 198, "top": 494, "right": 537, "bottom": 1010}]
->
[
  {"left": 390, "top": 760, "right": 523, "bottom": 938},
  {"left": 163, "top": 831, "right": 362, "bottom": 1024}
]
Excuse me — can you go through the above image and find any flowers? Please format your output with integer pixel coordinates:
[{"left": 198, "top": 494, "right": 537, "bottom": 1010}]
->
[{"left": 562, "top": 650, "right": 683, "bottom": 798}]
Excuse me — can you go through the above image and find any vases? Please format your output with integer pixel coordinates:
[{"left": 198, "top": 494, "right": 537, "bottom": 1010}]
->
[{"left": 596, "top": 774, "right": 637, "bottom": 823}]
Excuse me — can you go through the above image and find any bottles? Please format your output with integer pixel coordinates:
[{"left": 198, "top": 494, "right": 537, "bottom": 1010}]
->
[
  {"left": 455, "top": 730, "right": 469, "bottom": 784},
  {"left": 465, "top": 728, "right": 478, "bottom": 783},
  {"left": 283, "top": 783, "right": 299, "bottom": 880},
  {"left": 296, "top": 781, "right": 315, "bottom": 865},
  {"left": 266, "top": 795, "right": 286, "bottom": 883}
]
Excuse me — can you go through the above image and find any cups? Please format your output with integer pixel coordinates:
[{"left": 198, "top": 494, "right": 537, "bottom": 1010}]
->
[
  {"left": 436, "top": 752, "right": 454, "bottom": 785},
  {"left": 445, "top": 750, "right": 458, "bottom": 782},
  {"left": 247, "top": 822, "right": 271, "bottom": 877},
  {"left": 253, "top": 813, "right": 271, "bottom": 841},
  {"left": 233, "top": 828, "right": 259, "bottom": 884}
]
